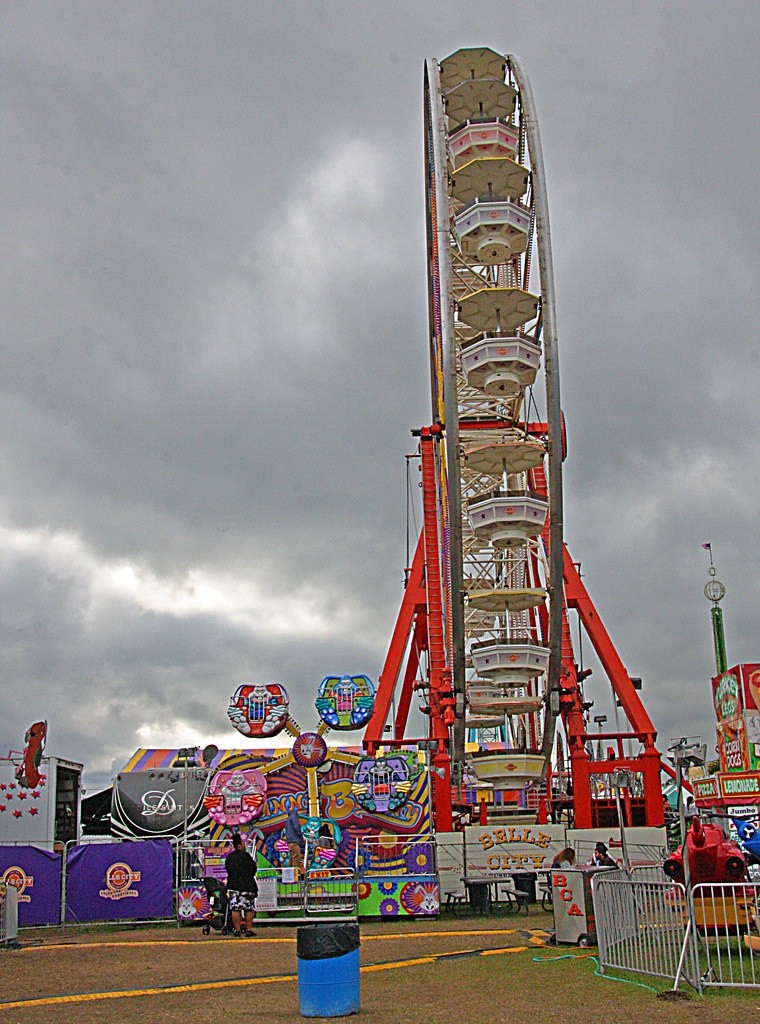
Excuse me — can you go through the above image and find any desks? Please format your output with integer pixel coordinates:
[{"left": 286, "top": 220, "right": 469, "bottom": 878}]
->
[{"left": 460, "top": 877, "right": 511, "bottom": 916}]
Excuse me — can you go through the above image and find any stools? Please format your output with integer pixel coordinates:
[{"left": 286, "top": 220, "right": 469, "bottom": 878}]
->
[
  {"left": 444, "top": 890, "right": 457, "bottom": 912},
  {"left": 452, "top": 894, "right": 465, "bottom": 918},
  {"left": 539, "top": 888, "right": 553, "bottom": 912},
  {"left": 501, "top": 890, "right": 529, "bottom": 916}
]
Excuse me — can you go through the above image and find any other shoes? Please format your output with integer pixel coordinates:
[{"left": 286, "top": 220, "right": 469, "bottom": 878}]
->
[
  {"left": 233, "top": 932, "right": 240, "bottom": 936},
  {"left": 246, "top": 930, "right": 257, "bottom": 937}
]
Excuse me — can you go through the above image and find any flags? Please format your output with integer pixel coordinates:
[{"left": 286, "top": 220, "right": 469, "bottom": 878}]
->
[{"left": 701, "top": 543, "right": 710, "bottom": 550}]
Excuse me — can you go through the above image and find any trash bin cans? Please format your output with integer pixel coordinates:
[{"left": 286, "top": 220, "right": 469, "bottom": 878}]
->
[
  {"left": 465, "top": 884, "right": 488, "bottom": 907},
  {"left": 510, "top": 871, "right": 538, "bottom": 902},
  {"left": 297, "top": 924, "right": 361, "bottom": 1017}
]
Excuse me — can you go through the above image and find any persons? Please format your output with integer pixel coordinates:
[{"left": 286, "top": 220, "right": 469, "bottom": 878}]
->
[
  {"left": 223, "top": 833, "right": 261, "bottom": 938},
  {"left": 662, "top": 791, "right": 673, "bottom": 812},
  {"left": 546, "top": 845, "right": 620, "bottom": 947},
  {"left": 684, "top": 794, "right": 699, "bottom": 814}
]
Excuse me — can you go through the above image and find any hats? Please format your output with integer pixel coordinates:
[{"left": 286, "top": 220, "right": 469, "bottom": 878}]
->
[{"left": 596, "top": 845, "right": 606, "bottom": 854}]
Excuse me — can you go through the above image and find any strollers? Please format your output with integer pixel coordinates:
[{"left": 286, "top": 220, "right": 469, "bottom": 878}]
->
[{"left": 197, "top": 876, "right": 246, "bottom": 936}]
